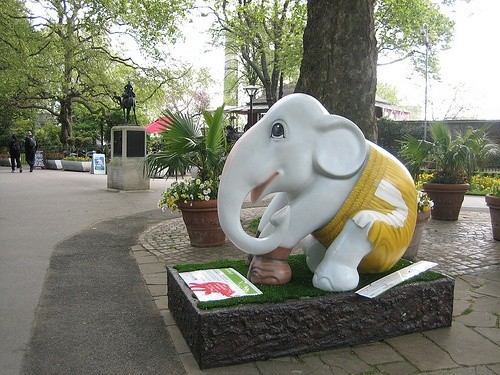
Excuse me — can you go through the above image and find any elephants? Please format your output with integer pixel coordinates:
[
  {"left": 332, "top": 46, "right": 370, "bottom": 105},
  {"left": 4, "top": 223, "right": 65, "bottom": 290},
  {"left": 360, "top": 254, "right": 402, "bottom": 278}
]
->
[{"left": 217, "top": 93, "right": 419, "bottom": 293}]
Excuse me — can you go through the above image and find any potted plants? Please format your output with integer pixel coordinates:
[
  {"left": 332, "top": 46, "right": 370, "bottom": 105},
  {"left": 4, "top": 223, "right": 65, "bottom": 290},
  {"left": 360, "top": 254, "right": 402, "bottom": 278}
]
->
[{"left": 398, "top": 120, "right": 500, "bottom": 221}]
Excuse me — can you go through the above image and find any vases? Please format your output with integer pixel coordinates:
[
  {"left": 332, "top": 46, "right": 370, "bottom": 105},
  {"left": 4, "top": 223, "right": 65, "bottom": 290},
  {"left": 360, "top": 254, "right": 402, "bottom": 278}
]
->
[
  {"left": 485, "top": 194, "right": 500, "bottom": 241},
  {"left": 44, "top": 159, "right": 92, "bottom": 172},
  {"left": 174, "top": 200, "right": 227, "bottom": 247},
  {"left": 0, "top": 157, "right": 21, "bottom": 167},
  {"left": 402, "top": 210, "right": 431, "bottom": 258}
]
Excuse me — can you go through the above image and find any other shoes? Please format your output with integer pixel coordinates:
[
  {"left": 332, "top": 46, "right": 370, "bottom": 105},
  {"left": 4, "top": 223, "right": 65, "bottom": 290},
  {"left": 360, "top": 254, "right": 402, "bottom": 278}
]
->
[
  {"left": 11, "top": 170, "right": 15, "bottom": 173},
  {"left": 20, "top": 170, "right": 22, "bottom": 173}
]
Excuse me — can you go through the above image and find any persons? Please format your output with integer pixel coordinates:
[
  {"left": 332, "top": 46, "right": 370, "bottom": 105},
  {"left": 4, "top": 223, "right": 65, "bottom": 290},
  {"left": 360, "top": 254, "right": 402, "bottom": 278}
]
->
[
  {"left": 225, "top": 125, "right": 240, "bottom": 143},
  {"left": 9, "top": 134, "right": 22, "bottom": 173},
  {"left": 124, "top": 80, "right": 136, "bottom": 97},
  {"left": 25, "top": 130, "right": 38, "bottom": 172}
]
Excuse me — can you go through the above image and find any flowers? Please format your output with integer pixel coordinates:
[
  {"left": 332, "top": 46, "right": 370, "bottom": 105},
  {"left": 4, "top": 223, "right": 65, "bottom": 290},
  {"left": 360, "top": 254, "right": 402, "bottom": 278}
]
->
[
  {"left": 414, "top": 190, "right": 435, "bottom": 213},
  {"left": 157, "top": 177, "right": 213, "bottom": 215}
]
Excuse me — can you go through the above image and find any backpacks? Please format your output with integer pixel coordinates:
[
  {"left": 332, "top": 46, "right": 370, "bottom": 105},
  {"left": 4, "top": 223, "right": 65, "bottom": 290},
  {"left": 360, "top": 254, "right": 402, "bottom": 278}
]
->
[{"left": 13, "top": 140, "right": 20, "bottom": 151}]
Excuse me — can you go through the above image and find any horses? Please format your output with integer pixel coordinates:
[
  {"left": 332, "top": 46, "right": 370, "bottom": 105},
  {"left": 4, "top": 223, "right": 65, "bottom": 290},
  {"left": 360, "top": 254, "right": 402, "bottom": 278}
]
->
[{"left": 114, "top": 96, "right": 140, "bottom": 126}]
